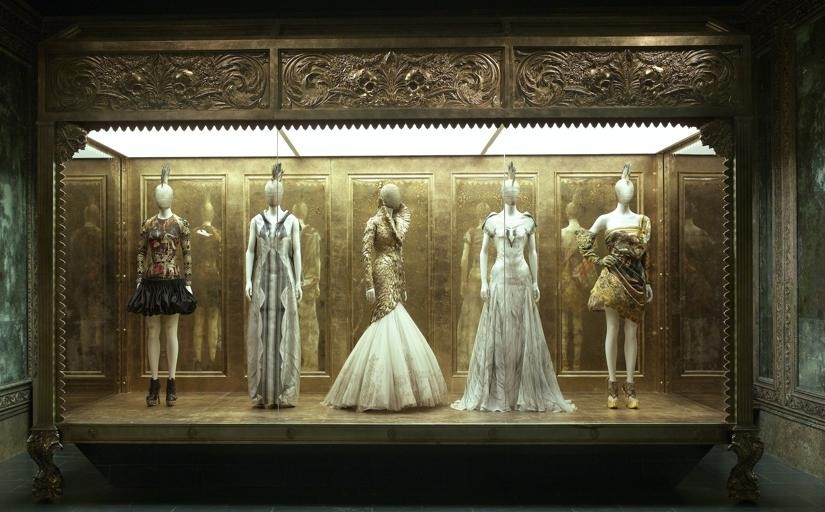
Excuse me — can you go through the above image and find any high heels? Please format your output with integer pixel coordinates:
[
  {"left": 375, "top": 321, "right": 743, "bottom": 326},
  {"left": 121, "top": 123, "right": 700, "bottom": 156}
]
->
[
  {"left": 165, "top": 377, "right": 178, "bottom": 407},
  {"left": 606, "top": 380, "right": 620, "bottom": 411},
  {"left": 254, "top": 393, "right": 267, "bottom": 409},
  {"left": 145, "top": 377, "right": 161, "bottom": 407},
  {"left": 192, "top": 359, "right": 205, "bottom": 370},
  {"left": 272, "top": 393, "right": 283, "bottom": 410},
  {"left": 207, "top": 359, "right": 217, "bottom": 370},
  {"left": 622, "top": 380, "right": 640, "bottom": 410}
]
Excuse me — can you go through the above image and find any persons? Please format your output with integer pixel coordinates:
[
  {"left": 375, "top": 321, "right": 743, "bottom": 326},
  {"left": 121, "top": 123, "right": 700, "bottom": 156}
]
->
[
  {"left": 243, "top": 162, "right": 304, "bottom": 409},
  {"left": 576, "top": 161, "right": 654, "bottom": 409},
  {"left": 319, "top": 184, "right": 452, "bottom": 413},
  {"left": 190, "top": 192, "right": 223, "bottom": 372},
  {"left": 449, "top": 160, "right": 578, "bottom": 414},
  {"left": 126, "top": 164, "right": 198, "bottom": 406},
  {"left": 452, "top": 193, "right": 497, "bottom": 372},
  {"left": 70, "top": 194, "right": 105, "bottom": 372},
  {"left": 291, "top": 190, "right": 323, "bottom": 374},
  {"left": 560, "top": 194, "right": 597, "bottom": 372}
]
